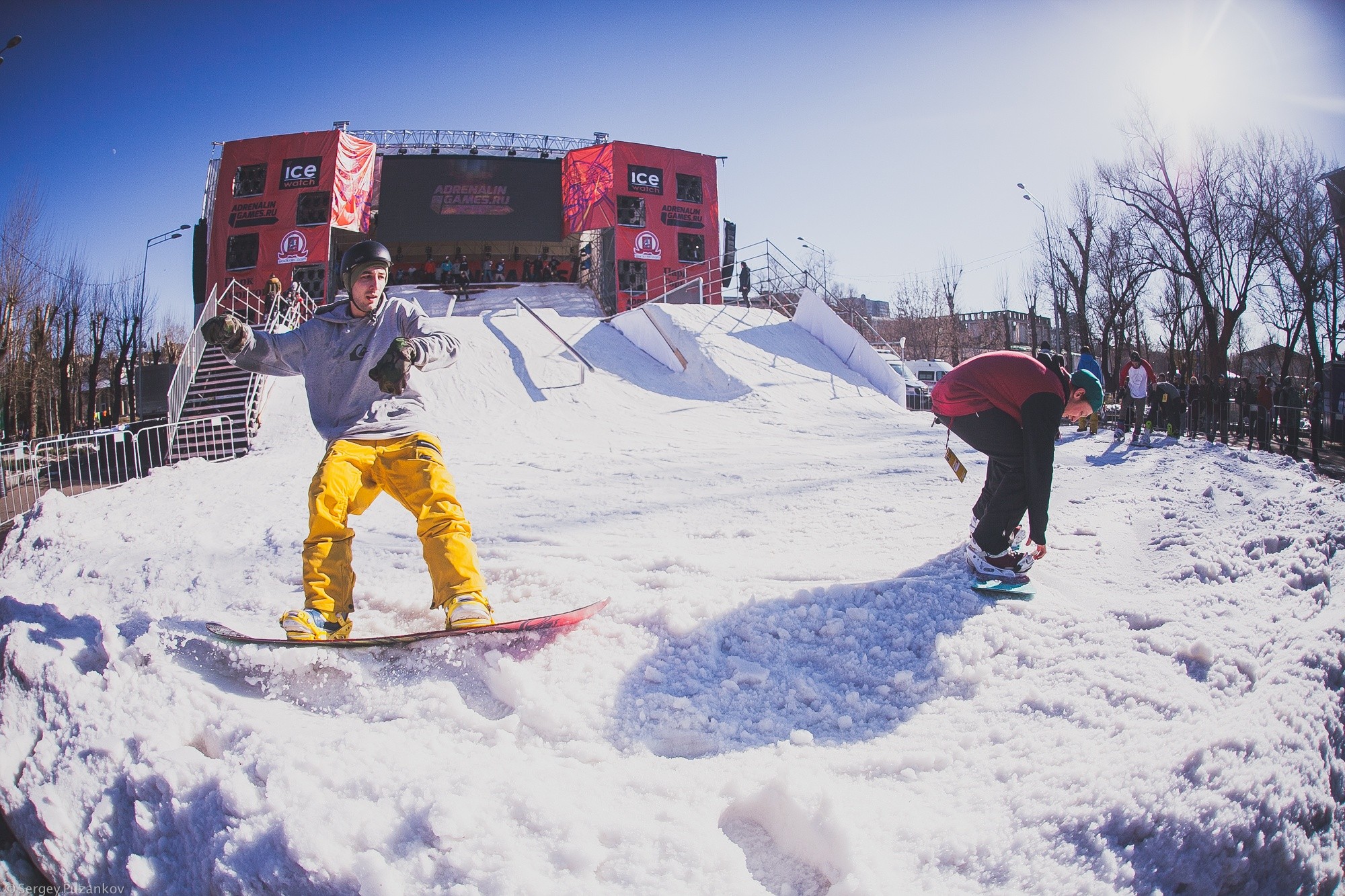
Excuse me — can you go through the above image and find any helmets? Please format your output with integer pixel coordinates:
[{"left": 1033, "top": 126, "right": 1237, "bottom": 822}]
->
[{"left": 341, "top": 241, "right": 393, "bottom": 288}]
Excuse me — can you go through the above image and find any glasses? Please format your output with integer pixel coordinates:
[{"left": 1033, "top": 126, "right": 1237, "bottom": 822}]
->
[
  {"left": 1173, "top": 377, "right": 1179, "bottom": 379},
  {"left": 1191, "top": 380, "right": 1196, "bottom": 383},
  {"left": 1219, "top": 379, "right": 1225, "bottom": 382}
]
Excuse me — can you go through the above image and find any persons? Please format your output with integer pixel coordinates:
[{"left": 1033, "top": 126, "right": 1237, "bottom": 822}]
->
[
  {"left": 930, "top": 351, "right": 1104, "bottom": 574},
  {"left": 261, "top": 272, "right": 282, "bottom": 323},
  {"left": 739, "top": 262, "right": 752, "bottom": 309},
  {"left": 1077, "top": 346, "right": 1104, "bottom": 435},
  {"left": 1121, "top": 369, "right": 1326, "bottom": 461},
  {"left": 1112, "top": 351, "right": 1157, "bottom": 442},
  {"left": 1035, "top": 341, "right": 1063, "bottom": 445},
  {"left": 386, "top": 253, "right": 567, "bottom": 302},
  {"left": 279, "top": 281, "right": 298, "bottom": 312},
  {"left": 277, "top": 291, "right": 294, "bottom": 323},
  {"left": 285, "top": 291, "right": 304, "bottom": 329},
  {"left": 200, "top": 240, "right": 495, "bottom": 644}
]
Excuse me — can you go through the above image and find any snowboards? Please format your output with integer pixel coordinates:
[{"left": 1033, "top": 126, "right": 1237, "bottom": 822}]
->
[
  {"left": 969, "top": 542, "right": 1034, "bottom": 596},
  {"left": 203, "top": 589, "right": 615, "bottom": 644}
]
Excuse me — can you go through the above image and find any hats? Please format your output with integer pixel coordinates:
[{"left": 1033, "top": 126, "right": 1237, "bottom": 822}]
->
[
  {"left": 1283, "top": 376, "right": 1291, "bottom": 385},
  {"left": 1070, "top": 369, "right": 1104, "bottom": 413},
  {"left": 1040, "top": 341, "right": 1051, "bottom": 351},
  {"left": 1131, "top": 352, "right": 1140, "bottom": 362},
  {"left": 1313, "top": 382, "right": 1321, "bottom": 390}
]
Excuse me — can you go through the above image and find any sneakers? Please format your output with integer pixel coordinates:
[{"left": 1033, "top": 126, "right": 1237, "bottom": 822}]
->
[
  {"left": 1088, "top": 432, "right": 1098, "bottom": 436},
  {"left": 967, "top": 538, "right": 1033, "bottom": 573},
  {"left": 1167, "top": 422, "right": 1176, "bottom": 437},
  {"left": 968, "top": 516, "right": 1027, "bottom": 542},
  {"left": 445, "top": 595, "right": 491, "bottom": 622},
  {"left": 1146, "top": 420, "right": 1153, "bottom": 433},
  {"left": 283, "top": 608, "right": 348, "bottom": 634},
  {"left": 1077, "top": 427, "right": 1087, "bottom": 432},
  {"left": 1134, "top": 428, "right": 1142, "bottom": 435},
  {"left": 1114, "top": 429, "right": 1124, "bottom": 437}
]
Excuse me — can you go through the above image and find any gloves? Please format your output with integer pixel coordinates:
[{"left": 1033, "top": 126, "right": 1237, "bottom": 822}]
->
[
  {"left": 201, "top": 313, "right": 249, "bottom": 353},
  {"left": 368, "top": 337, "right": 417, "bottom": 397}
]
